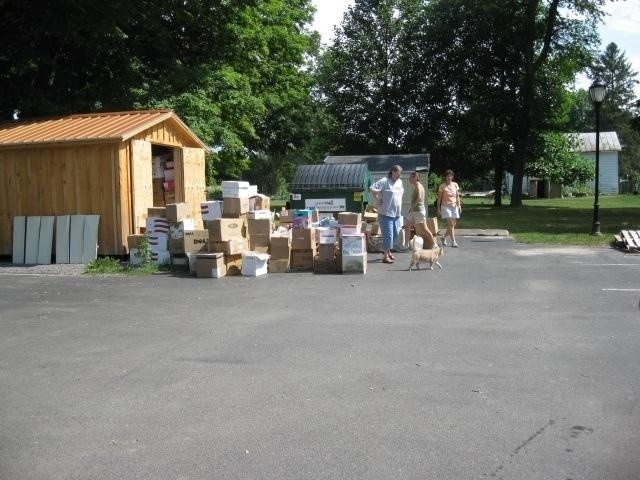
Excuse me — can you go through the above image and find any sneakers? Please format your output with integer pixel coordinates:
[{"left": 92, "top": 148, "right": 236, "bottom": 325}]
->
[
  {"left": 450, "top": 240, "right": 458, "bottom": 248},
  {"left": 440, "top": 236, "right": 447, "bottom": 246},
  {"left": 431, "top": 244, "right": 438, "bottom": 249}
]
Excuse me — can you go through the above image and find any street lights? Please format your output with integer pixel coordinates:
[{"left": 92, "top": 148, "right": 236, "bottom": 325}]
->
[{"left": 588, "top": 76, "right": 607, "bottom": 236}]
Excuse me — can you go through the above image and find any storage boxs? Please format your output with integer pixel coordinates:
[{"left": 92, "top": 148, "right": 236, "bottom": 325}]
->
[{"left": 126, "top": 154, "right": 439, "bottom": 280}]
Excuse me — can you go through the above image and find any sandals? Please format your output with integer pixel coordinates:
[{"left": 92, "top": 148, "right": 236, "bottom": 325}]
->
[{"left": 383, "top": 254, "right": 395, "bottom": 264}]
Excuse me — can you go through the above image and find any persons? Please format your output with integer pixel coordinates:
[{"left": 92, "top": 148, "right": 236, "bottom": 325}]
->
[
  {"left": 369, "top": 164, "right": 404, "bottom": 263},
  {"left": 405, "top": 172, "right": 439, "bottom": 250},
  {"left": 436, "top": 169, "right": 462, "bottom": 247}
]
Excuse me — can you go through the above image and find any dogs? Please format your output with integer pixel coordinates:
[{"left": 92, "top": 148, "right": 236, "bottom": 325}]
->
[{"left": 408, "top": 235, "right": 444, "bottom": 273}]
